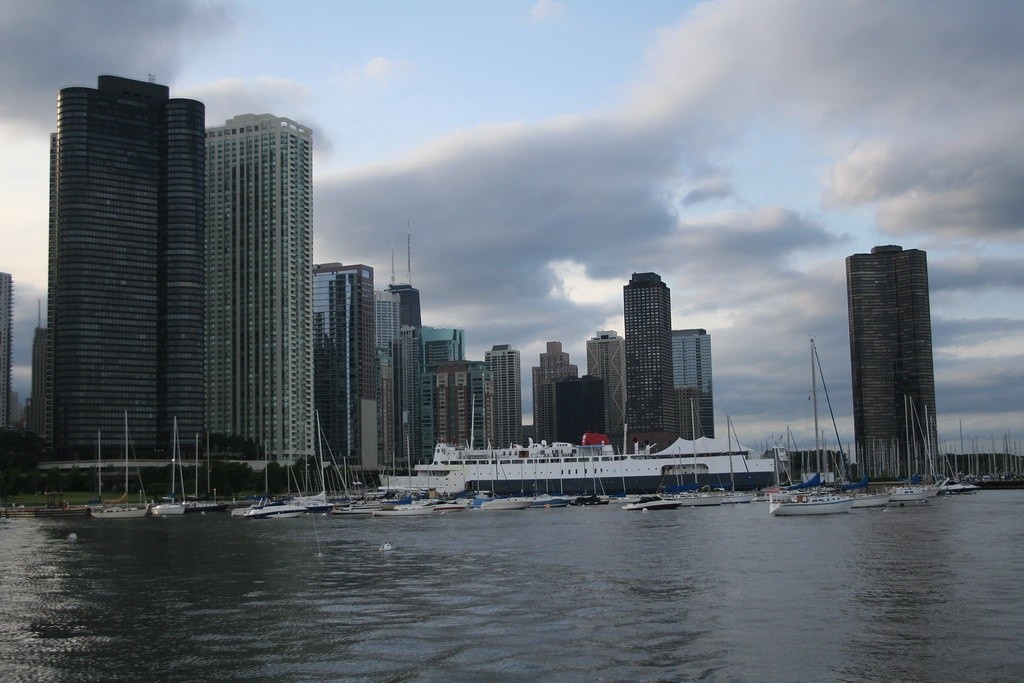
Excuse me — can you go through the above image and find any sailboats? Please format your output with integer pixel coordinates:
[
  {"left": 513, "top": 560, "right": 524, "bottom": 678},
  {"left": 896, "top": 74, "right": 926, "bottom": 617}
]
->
[{"left": 1, "top": 335, "right": 1024, "bottom": 515}]
